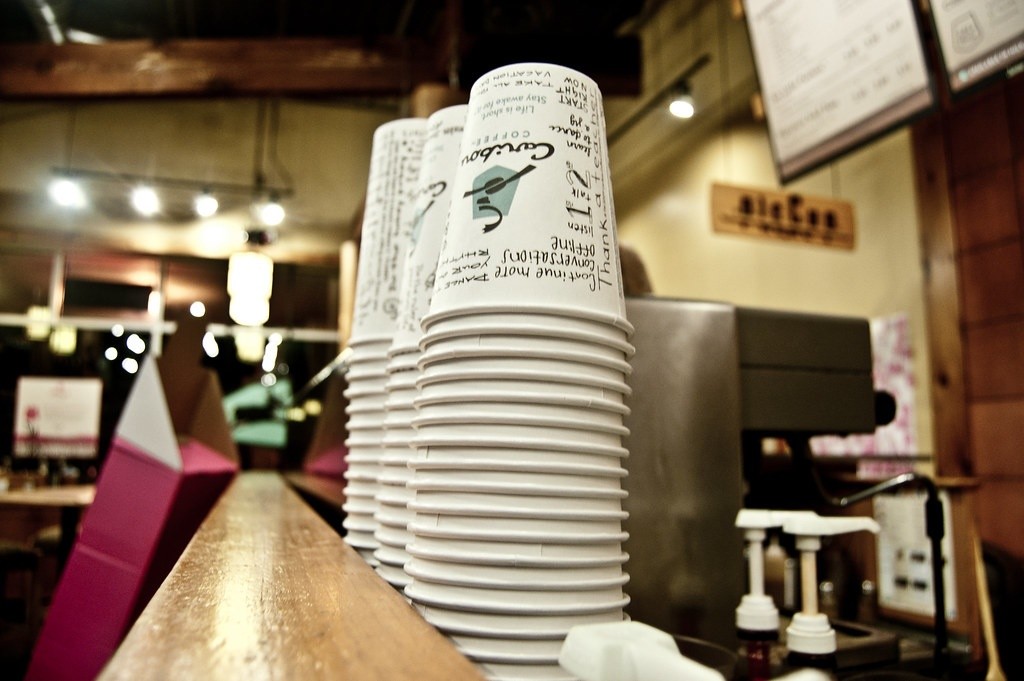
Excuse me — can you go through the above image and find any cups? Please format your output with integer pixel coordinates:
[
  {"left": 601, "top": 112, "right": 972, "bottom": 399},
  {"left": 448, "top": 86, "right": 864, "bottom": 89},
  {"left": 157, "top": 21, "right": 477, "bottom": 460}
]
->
[{"left": 343, "top": 62, "right": 633, "bottom": 664}]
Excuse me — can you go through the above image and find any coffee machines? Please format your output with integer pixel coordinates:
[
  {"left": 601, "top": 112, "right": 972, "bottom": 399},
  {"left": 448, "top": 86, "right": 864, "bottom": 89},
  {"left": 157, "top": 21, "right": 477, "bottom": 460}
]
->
[{"left": 622, "top": 297, "right": 901, "bottom": 678}]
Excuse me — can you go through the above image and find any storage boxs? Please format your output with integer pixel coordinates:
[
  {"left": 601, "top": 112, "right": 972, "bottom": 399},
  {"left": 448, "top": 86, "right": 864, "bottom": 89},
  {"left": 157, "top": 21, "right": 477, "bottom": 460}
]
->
[{"left": 26, "top": 354, "right": 241, "bottom": 680}]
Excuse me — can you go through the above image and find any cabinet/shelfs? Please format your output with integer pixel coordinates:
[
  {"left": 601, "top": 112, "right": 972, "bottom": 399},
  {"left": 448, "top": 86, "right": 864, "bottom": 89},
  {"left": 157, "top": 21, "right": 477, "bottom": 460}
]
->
[{"left": 828, "top": 467, "right": 987, "bottom": 667}]
